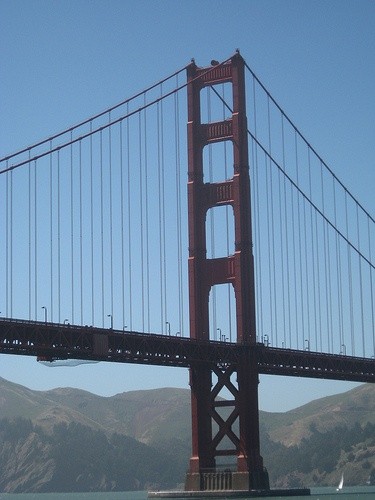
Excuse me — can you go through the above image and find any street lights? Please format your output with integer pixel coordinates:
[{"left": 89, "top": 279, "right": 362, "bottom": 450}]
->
[
  {"left": 217, "top": 328, "right": 221, "bottom": 341},
  {"left": 107, "top": 315, "right": 113, "bottom": 329},
  {"left": 42, "top": 306, "right": 47, "bottom": 323},
  {"left": 222, "top": 334, "right": 347, "bottom": 356},
  {"left": 176, "top": 332, "right": 180, "bottom": 336},
  {"left": 165, "top": 322, "right": 170, "bottom": 336},
  {"left": 123, "top": 326, "right": 127, "bottom": 331},
  {"left": 64, "top": 319, "right": 68, "bottom": 324}
]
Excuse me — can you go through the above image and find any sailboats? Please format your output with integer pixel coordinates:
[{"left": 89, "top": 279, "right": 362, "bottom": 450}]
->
[{"left": 336, "top": 473, "right": 344, "bottom": 492}]
